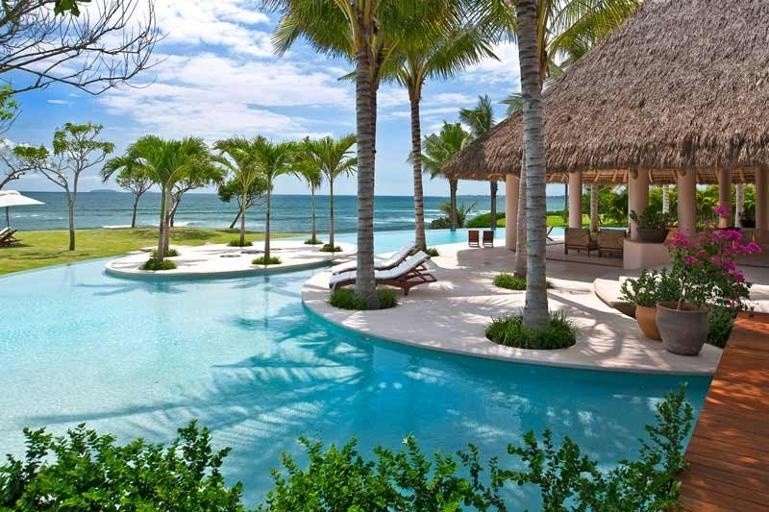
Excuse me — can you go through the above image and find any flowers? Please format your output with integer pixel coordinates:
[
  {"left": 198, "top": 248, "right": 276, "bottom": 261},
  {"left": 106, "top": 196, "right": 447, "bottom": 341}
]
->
[{"left": 666, "top": 229, "right": 711, "bottom": 310}]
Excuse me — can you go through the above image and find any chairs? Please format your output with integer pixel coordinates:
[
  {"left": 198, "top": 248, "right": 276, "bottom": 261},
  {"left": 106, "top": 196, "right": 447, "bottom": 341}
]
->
[
  {"left": 564, "top": 228, "right": 597, "bottom": 256},
  {"left": 331, "top": 241, "right": 427, "bottom": 276},
  {"left": 483, "top": 231, "right": 493, "bottom": 248},
  {"left": 329, "top": 250, "right": 437, "bottom": 295},
  {"left": 0, "top": 229, "right": 18, "bottom": 246},
  {"left": 468, "top": 230, "right": 479, "bottom": 247},
  {"left": 0, "top": 226, "right": 11, "bottom": 235},
  {"left": 597, "top": 231, "right": 626, "bottom": 257}
]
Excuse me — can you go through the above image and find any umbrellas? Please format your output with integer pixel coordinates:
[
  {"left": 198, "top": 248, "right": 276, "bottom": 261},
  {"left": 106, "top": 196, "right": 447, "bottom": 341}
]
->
[{"left": 0, "top": 190, "right": 46, "bottom": 241}]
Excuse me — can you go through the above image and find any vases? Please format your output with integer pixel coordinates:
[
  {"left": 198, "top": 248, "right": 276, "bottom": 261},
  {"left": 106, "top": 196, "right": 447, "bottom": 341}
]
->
[{"left": 656, "top": 302, "right": 709, "bottom": 356}]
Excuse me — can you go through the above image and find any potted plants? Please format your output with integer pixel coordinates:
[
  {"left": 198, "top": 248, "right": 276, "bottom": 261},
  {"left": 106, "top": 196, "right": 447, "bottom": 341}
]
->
[
  {"left": 629, "top": 205, "right": 672, "bottom": 242},
  {"left": 617, "top": 265, "right": 682, "bottom": 340}
]
